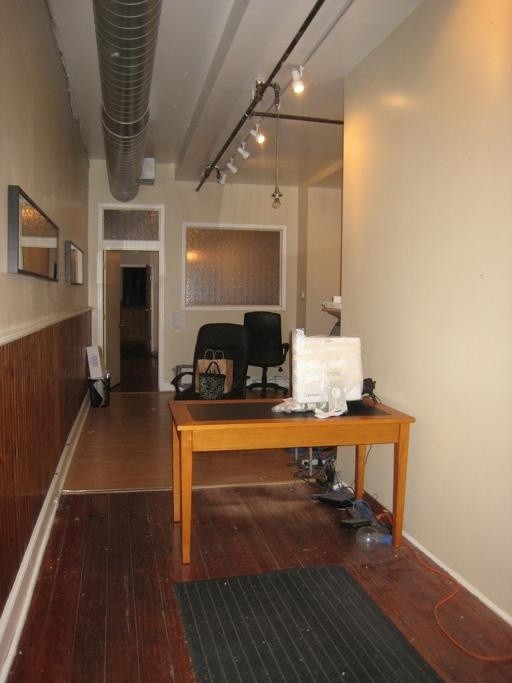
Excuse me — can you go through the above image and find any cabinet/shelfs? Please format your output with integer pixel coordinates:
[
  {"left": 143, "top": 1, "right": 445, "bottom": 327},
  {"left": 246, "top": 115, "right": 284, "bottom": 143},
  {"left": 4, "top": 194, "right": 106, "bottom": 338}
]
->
[
  {"left": 120, "top": 263, "right": 151, "bottom": 307},
  {"left": 120, "top": 304, "right": 151, "bottom": 349}
]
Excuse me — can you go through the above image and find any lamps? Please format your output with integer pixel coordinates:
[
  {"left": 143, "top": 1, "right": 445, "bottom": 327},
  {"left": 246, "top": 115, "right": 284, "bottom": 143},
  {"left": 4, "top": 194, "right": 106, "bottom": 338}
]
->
[
  {"left": 272, "top": 83, "right": 283, "bottom": 209},
  {"left": 218, "top": 64, "right": 305, "bottom": 185}
]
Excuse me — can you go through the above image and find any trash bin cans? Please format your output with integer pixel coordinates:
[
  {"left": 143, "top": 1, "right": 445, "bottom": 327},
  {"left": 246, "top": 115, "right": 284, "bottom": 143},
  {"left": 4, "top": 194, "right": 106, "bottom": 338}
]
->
[{"left": 88, "top": 378, "right": 109, "bottom": 407}]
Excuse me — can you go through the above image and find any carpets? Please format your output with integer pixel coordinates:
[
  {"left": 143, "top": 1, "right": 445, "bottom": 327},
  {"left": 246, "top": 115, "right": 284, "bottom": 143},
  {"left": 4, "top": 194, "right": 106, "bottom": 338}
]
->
[{"left": 172, "top": 564, "right": 445, "bottom": 683}]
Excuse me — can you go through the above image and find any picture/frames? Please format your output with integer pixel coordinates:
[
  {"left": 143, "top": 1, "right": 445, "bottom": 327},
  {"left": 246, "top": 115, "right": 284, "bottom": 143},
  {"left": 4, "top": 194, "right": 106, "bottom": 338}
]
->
[
  {"left": 65, "top": 240, "right": 84, "bottom": 286},
  {"left": 8, "top": 184, "right": 59, "bottom": 282}
]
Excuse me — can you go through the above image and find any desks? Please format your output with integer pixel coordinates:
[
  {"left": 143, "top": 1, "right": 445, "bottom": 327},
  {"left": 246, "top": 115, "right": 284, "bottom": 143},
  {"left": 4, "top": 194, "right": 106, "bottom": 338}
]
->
[{"left": 168, "top": 398, "right": 416, "bottom": 564}]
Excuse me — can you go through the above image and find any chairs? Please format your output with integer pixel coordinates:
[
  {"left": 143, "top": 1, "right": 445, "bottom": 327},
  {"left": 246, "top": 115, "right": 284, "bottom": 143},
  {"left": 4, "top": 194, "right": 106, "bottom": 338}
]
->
[
  {"left": 171, "top": 322, "right": 250, "bottom": 400},
  {"left": 244, "top": 311, "right": 289, "bottom": 397}
]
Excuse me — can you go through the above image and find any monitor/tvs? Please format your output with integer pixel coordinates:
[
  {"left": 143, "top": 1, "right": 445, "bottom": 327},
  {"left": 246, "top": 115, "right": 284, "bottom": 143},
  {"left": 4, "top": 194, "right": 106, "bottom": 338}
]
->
[{"left": 291, "top": 336, "right": 364, "bottom": 414}]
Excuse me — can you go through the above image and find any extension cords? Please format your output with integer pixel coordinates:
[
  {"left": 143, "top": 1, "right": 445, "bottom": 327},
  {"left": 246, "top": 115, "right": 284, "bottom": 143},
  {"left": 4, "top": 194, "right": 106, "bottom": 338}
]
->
[{"left": 302, "top": 459, "right": 326, "bottom": 466}]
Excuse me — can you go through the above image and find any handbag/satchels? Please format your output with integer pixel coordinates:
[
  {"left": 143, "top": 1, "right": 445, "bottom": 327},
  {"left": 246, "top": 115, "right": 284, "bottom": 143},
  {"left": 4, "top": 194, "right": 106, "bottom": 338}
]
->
[{"left": 194, "top": 348, "right": 234, "bottom": 400}]
